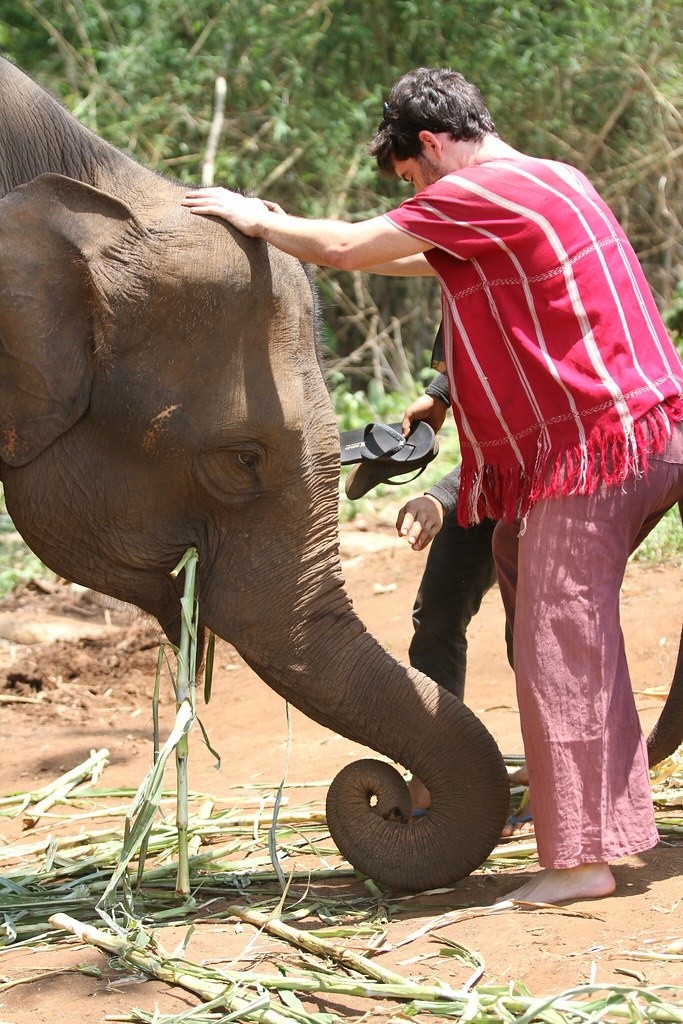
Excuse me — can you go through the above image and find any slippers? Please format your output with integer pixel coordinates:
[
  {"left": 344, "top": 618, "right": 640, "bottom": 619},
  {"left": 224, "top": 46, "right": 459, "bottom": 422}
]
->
[
  {"left": 345, "top": 440, "right": 440, "bottom": 501},
  {"left": 500, "top": 815, "right": 537, "bottom": 842},
  {"left": 410, "top": 808, "right": 429, "bottom": 825},
  {"left": 340, "top": 420, "right": 436, "bottom": 463}
]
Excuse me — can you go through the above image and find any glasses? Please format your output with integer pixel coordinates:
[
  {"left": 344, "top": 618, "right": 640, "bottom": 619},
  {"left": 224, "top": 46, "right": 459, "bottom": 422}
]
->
[{"left": 382, "top": 101, "right": 430, "bottom": 129}]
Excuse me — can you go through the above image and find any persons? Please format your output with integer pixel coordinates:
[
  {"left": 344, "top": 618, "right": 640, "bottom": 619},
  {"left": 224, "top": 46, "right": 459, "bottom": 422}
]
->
[
  {"left": 181, "top": 66, "right": 683, "bottom": 909},
  {"left": 397, "top": 322, "right": 536, "bottom": 843}
]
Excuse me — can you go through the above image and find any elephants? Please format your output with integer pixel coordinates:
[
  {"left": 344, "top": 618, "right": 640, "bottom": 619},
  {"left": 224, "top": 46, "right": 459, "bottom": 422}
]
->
[{"left": 0, "top": 56, "right": 510, "bottom": 895}]
